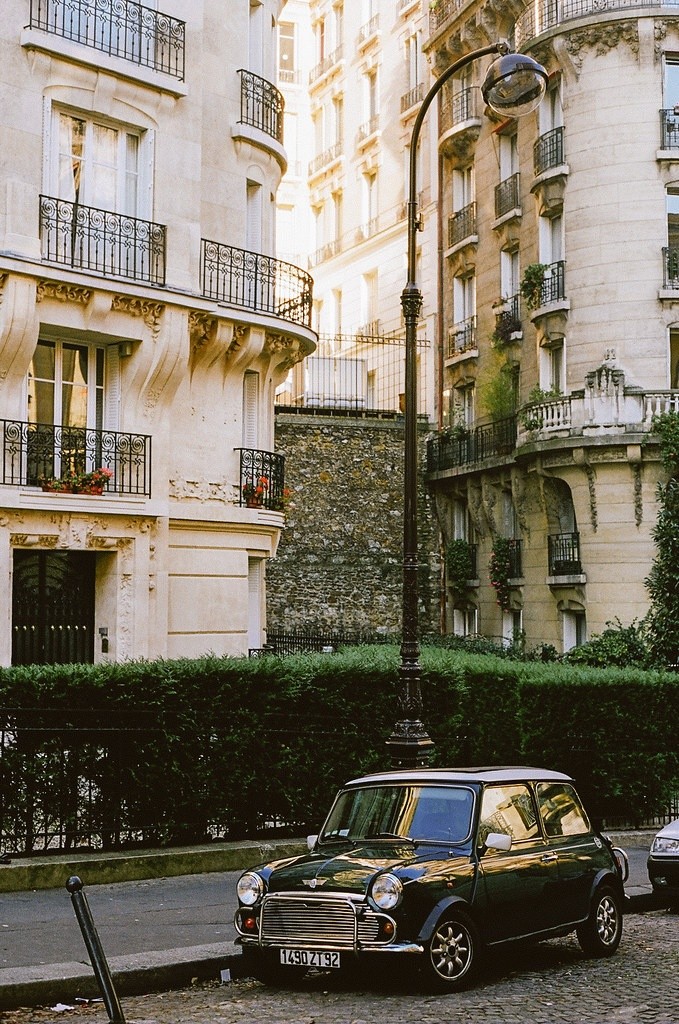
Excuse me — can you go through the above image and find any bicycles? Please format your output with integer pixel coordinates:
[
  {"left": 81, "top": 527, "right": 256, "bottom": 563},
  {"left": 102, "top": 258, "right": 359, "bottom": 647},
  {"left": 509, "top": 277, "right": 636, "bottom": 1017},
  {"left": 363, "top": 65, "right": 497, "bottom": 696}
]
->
[{"left": 606, "top": 837, "right": 630, "bottom": 884}]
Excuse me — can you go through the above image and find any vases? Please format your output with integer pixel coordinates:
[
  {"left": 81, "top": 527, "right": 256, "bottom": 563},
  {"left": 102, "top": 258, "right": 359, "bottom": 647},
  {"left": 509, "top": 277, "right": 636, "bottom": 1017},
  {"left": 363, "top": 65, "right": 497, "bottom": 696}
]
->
[
  {"left": 247, "top": 495, "right": 261, "bottom": 509},
  {"left": 42, "top": 483, "right": 104, "bottom": 496}
]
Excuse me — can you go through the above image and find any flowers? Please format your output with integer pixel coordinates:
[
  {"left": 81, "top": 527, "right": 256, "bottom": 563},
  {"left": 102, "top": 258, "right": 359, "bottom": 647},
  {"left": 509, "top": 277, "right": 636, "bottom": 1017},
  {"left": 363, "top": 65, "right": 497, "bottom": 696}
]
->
[
  {"left": 38, "top": 466, "right": 112, "bottom": 494},
  {"left": 268, "top": 488, "right": 290, "bottom": 510},
  {"left": 489, "top": 536, "right": 510, "bottom": 613},
  {"left": 242, "top": 476, "right": 268, "bottom": 499}
]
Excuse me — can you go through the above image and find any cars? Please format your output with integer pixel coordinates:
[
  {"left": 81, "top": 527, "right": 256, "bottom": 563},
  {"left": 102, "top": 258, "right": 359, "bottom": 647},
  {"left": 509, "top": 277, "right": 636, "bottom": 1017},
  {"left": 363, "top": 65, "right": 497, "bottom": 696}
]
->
[
  {"left": 647, "top": 819, "right": 679, "bottom": 915},
  {"left": 231, "top": 765, "right": 630, "bottom": 991}
]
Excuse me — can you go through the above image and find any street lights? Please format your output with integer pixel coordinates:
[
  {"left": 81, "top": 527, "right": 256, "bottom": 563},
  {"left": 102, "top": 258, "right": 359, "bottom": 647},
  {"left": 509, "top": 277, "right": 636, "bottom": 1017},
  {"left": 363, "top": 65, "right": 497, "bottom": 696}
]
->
[{"left": 385, "top": 37, "right": 548, "bottom": 764}]
